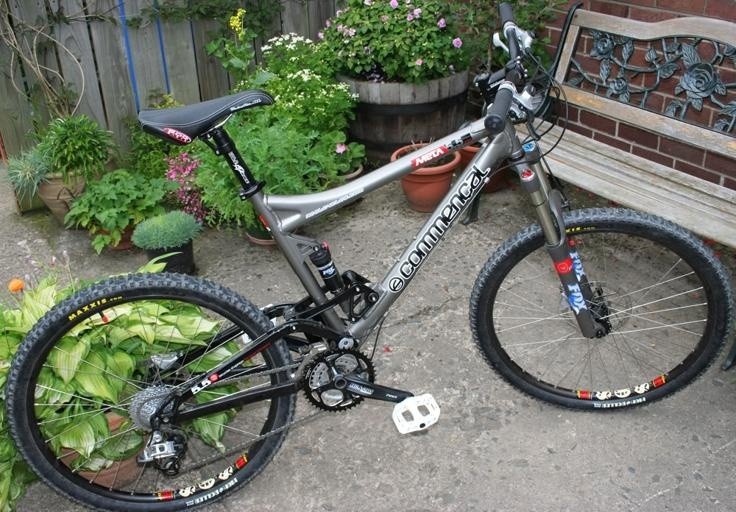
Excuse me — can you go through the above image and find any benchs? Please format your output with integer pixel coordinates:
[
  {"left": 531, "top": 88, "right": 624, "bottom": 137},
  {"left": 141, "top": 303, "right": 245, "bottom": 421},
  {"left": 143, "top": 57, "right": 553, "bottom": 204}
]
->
[{"left": 459, "top": 1, "right": 736, "bottom": 370}]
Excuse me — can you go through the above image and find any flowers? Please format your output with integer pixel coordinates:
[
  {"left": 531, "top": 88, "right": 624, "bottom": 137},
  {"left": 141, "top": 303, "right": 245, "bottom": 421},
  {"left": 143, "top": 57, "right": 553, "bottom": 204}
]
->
[
  {"left": 307, "top": 130, "right": 364, "bottom": 176},
  {"left": 318, "top": 0, "right": 480, "bottom": 87}
]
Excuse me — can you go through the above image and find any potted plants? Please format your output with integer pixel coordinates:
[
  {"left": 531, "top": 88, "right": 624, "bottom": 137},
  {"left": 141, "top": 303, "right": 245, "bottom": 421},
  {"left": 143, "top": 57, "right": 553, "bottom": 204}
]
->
[
  {"left": 0, "top": 252, "right": 255, "bottom": 512},
  {"left": 131, "top": 210, "right": 204, "bottom": 275},
  {"left": 196, "top": 124, "right": 338, "bottom": 239},
  {"left": 63, "top": 170, "right": 180, "bottom": 255},
  {"left": 4, "top": 113, "right": 122, "bottom": 229}
]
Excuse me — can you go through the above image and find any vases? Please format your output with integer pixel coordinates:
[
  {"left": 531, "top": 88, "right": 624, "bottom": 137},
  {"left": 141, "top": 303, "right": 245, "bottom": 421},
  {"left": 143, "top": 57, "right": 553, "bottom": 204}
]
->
[
  {"left": 392, "top": 142, "right": 461, "bottom": 212},
  {"left": 309, "top": 160, "right": 364, "bottom": 188},
  {"left": 335, "top": 65, "right": 470, "bottom": 159},
  {"left": 459, "top": 146, "right": 505, "bottom": 192}
]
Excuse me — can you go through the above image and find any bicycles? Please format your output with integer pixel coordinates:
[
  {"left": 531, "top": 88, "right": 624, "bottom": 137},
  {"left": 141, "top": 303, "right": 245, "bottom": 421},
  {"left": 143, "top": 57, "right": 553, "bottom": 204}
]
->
[{"left": 5, "top": 2, "right": 730, "bottom": 511}]
